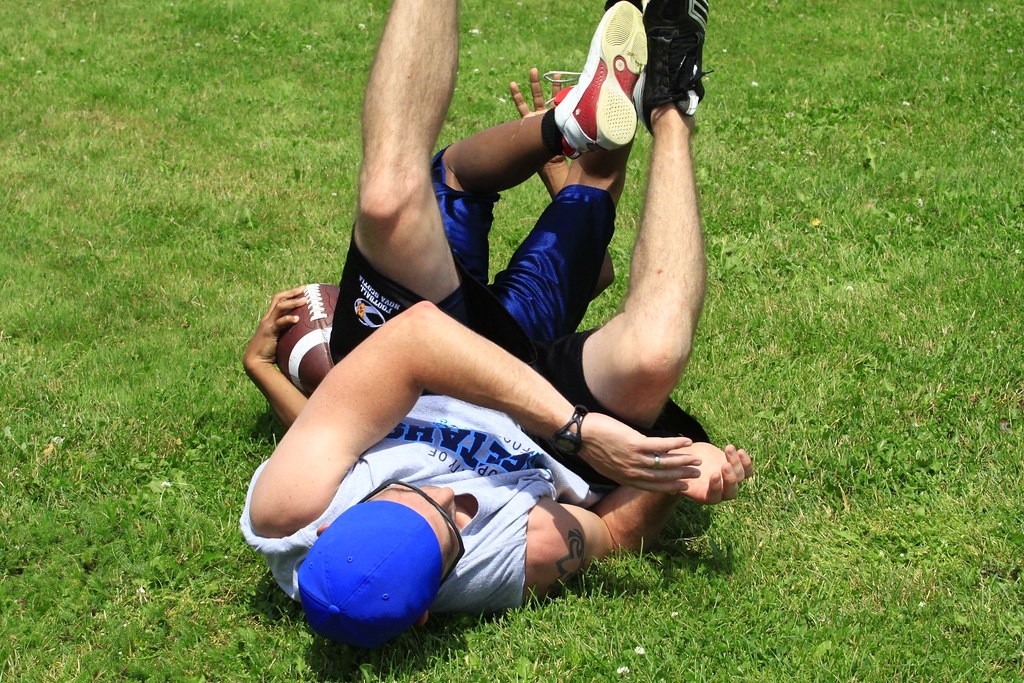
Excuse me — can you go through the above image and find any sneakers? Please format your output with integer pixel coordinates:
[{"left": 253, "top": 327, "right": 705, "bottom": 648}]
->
[
  {"left": 636, "top": 0, "right": 708, "bottom": 132},
  {"left": 554, "top": 0, "right": 649, "bottom": 158}
]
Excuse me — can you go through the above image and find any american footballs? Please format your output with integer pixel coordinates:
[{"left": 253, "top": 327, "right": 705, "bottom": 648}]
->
[{"left": 276, "top": 283, "right": 341, "bottom": 396}]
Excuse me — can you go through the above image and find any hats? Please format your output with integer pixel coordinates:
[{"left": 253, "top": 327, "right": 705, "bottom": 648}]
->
[{"left": 302, "top": 499, "right": 444, "bottom": 649}]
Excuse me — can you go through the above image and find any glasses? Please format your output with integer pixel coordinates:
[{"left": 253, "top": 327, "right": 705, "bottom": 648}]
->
[{"left": 356, "top": 478, "right": 466, "bottom": 590}]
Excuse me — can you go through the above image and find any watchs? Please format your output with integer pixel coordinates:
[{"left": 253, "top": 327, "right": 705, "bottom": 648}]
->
[{"left": 550, "top": 405, "right": 590, "bottom": 461}]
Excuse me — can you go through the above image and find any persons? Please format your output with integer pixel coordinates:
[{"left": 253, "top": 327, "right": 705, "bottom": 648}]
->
[
  {"left": 239, "top": 0, "right": 758, "bottom": 650},
  {"left": 245, "top": 0, "right": 651, "bottom": 427}
]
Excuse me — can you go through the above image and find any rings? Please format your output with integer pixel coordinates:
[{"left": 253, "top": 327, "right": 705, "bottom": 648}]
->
[{"left": 652, "top": 452, "right": 660, "bottom": 469}]
